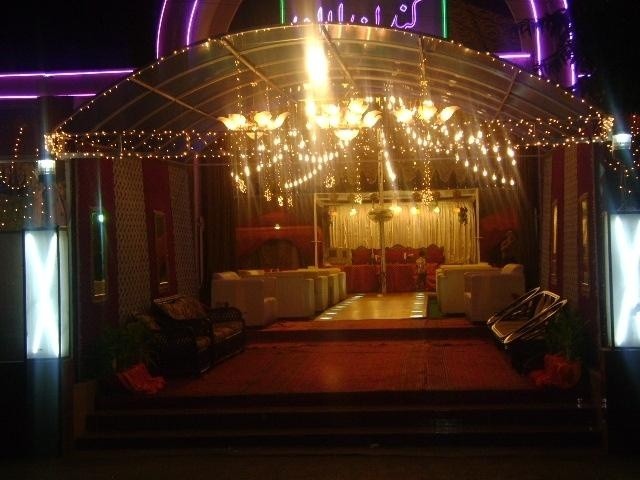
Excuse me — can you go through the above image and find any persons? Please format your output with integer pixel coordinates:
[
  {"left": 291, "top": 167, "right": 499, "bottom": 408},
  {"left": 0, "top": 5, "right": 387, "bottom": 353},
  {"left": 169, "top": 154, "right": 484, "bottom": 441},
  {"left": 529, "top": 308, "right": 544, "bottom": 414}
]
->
[
  {"left": 415, "top": 250, "right": 429, "bottom": 291},
  {"left": 373, "top": 256, "right": 384, "bottom": 292},
  {"left": 500, "top": 229, "right": 519, "bottom": 267}
]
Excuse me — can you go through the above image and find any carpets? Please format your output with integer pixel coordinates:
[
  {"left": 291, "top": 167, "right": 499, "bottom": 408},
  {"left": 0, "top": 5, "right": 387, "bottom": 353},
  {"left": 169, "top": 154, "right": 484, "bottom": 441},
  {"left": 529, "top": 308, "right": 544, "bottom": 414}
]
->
[{"left": 313, "top": 290, "right": 429, "bottom": 321}]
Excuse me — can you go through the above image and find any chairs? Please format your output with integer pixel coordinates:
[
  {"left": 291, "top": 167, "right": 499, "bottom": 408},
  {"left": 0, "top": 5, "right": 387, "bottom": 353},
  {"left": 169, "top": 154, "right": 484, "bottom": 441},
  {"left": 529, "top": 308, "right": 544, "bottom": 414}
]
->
[
  {"left": 351, "top": 245, "right": 444, "bottom": 265},
  {"left": 487, "top": 286, "right": 569, "bottom": 375},
  {"left": 132, "top": 310, "right": 217, "bottom": 377}
]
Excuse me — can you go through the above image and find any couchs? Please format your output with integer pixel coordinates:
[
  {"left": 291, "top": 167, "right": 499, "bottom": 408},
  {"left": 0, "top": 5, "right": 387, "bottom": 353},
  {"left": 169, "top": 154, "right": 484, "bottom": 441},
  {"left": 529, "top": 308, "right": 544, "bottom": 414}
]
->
[
  {"left": 209, "top": 269, "right": 346, "bottom": 328},
  {"left": 148, "top": 294, "right": 248, "bottom": 364},
  {"left": 434, "top": 263, "right": 525, "bottom": 325}
]
